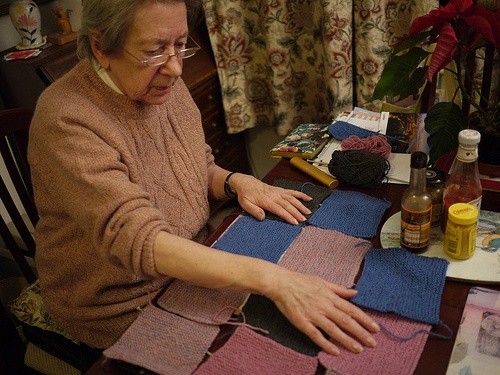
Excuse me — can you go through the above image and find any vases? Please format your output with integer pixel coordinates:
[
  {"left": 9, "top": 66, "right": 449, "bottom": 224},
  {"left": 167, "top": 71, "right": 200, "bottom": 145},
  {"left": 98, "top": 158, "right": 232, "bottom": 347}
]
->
[{"left": 469, "top": 110, "right": 500, "bottom": 167}]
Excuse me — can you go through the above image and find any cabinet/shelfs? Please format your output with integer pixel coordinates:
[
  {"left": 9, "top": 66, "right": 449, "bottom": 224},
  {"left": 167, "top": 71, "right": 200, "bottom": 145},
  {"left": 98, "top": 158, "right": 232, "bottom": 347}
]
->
[{"left": 0, "top": 31, "right": 253, "bottom": 212}]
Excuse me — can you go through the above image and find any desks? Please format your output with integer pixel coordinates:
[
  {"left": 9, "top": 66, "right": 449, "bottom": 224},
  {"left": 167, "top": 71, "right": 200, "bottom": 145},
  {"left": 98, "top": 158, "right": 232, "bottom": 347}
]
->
[{"left": 84, "top": 158, "right": 500, "bottom": 374}]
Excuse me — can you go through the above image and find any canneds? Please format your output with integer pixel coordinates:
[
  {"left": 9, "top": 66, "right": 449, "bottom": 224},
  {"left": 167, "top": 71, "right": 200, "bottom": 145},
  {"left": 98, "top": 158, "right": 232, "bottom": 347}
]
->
[{"left": 443, "top": 202, "right": 479, "bottom": 259}]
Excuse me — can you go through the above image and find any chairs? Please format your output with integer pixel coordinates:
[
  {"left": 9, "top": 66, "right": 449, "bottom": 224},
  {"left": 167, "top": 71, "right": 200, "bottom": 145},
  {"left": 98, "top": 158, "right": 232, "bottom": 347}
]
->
[{"left": 1, "top": 117, "right": 103, "bottom": 374}]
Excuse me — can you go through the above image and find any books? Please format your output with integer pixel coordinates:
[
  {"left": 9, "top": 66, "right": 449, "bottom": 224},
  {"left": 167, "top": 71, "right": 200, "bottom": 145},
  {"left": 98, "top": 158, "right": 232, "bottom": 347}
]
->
[{"left": 270, "top": 123, "right": 332, "bottom": 160}]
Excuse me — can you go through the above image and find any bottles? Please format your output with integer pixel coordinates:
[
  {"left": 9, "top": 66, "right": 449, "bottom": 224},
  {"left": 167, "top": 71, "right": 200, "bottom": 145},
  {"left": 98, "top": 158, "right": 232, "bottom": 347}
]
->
[
  {"left": 440, "top": 128, "right": 483, "bottom": 235},
  {"left": 8, "top": 0, "right": 42, "bottom": 47},
  {"left": 443, "top": 203, "right": 479, "bottom": 260},
  {"left": 400, "top": 151, "right": 433, "bottom": 254}
]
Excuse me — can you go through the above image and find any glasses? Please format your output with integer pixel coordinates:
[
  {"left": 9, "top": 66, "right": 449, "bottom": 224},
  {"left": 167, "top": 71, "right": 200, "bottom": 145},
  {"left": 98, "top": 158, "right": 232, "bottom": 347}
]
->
[{"left": 114, "top": 35, "right": 201, "bottom": 66}]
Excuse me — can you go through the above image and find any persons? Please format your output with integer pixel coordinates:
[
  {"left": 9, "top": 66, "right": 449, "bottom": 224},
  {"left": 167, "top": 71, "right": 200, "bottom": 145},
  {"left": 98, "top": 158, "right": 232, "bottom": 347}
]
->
[{"left": 26, "top": 0, "right": 381, "bottom": 355}]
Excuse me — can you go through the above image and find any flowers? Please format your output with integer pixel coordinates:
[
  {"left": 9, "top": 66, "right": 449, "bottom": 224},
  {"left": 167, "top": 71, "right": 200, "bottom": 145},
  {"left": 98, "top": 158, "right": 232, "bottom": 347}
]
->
[{"left": 373, "top": 0, "right": 500, "bottom": 159}]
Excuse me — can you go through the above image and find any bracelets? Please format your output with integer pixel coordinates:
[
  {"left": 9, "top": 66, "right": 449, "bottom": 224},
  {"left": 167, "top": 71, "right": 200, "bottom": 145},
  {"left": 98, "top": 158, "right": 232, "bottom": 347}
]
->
[{"left": 222, "top": 172, "right": 237, "bottom": 199}]
